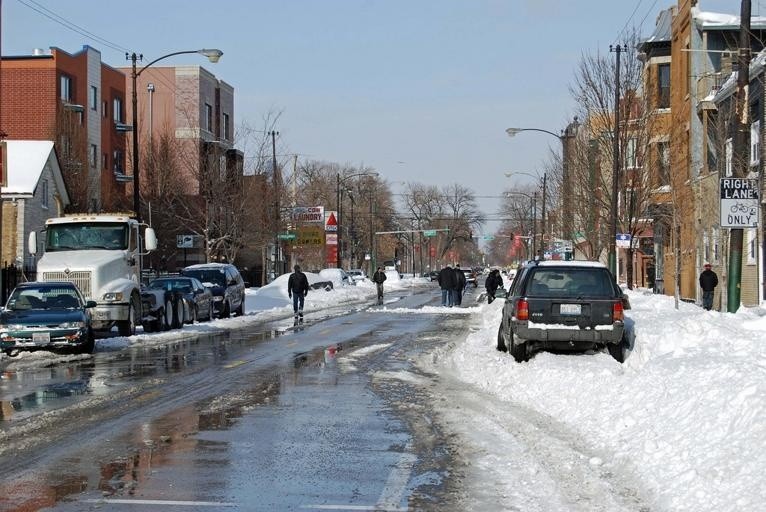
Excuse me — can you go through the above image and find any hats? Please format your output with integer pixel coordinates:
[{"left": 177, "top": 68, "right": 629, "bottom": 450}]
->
[{"left": 704, "top": 264, "right": 712, "bottom": 268}]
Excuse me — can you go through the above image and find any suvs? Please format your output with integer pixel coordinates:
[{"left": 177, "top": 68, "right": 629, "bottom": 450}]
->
[{"left": 496, "top": 259, "right": 632, "bottom": 363}]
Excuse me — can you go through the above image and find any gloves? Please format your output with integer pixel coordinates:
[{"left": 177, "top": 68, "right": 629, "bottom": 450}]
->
[
  {"left": 304, "top": 291, "right": 308, "bottom": 296},
  {"left": 288, "top": 293, "right": 292, "bottom": 299}
]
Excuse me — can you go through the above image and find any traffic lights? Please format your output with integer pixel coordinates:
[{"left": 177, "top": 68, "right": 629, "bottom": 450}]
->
[{"left": 470, "top": 233, "right": 472, "bottom": 239}]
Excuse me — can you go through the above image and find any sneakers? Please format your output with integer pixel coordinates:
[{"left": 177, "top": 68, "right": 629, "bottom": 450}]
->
[
  {"left": 442, "top": 303, "right": 461, "bottom": 308},
  {"left": 294, "top": 312, "right": 298, "bottom": 319},
  {"left": 299, "top": 310, "right": 303, "bottom": 317}
]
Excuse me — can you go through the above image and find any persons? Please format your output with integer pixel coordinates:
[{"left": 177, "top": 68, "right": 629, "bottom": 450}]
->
[
  {"left": 699, "top": 263, "right": 718, "bottom": 311},
  {"left": 373, "top": 266, "right": 387, "bottom": 299},
  {"left": 485, "top": 269, "right": 503, "bottom": 304},
  {"left": 452, "top": 264, "right": 466, "bottom": 307},
  {"left": 438, "top": 263, "right": 457, "bottom": 308},
  {"left": 288, "top": 264, "right": 309, "bottom": 318}
]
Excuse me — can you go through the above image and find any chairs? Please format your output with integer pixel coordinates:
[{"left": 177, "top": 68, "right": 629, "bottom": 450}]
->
[
  {"left": 534, "top": 279, "right": 593, "bottom": 292},
  {"left": 57, "top": 294, "right": 74, "bottom": 307},
  {"left": 15, "top": 295, "right": 33, "bottom": 309}
]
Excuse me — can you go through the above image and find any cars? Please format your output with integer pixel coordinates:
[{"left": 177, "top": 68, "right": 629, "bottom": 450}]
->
[
  {"left": 278, "top": 268, "right": 367, "bottom": 293},
  {"left": 423, "top": 264, "right": 485, "bottom": 286},
  {"left": 149, "top": 276, "right": 214, "bottom": 322},
  {"left": 1, "top": 281, "right": 97, "bottom": 358}
]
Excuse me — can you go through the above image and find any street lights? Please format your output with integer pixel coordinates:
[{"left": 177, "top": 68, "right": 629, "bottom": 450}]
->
[
  {"left": 336, "top": 171, "right": 380, "bottom": 268},
  {"left": 124, "top": 48, "right": 224, "bottom": 227},
  {"left": 504, "top": 169, "right": 548, "bottom": 263},
  {"left": 505, "top": 123, "right": 573, "bottom": 263}
]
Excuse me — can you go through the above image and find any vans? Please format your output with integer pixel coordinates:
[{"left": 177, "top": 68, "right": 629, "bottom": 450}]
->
[{"left": 179, "top": 261, "right": 247, "bottom": 317}]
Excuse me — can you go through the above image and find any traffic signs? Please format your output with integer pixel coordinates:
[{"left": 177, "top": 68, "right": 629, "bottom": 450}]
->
[
  {"left": 424, "top": 230, "right": 436, "bottom": 237},
  {"left": 276, "top": 233, "right": 297, "bottom": 240}
]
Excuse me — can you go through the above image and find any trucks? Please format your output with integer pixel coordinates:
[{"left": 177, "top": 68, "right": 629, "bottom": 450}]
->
[{"left": 27, "top": 211, "right": 184, "bottom": 338}]
